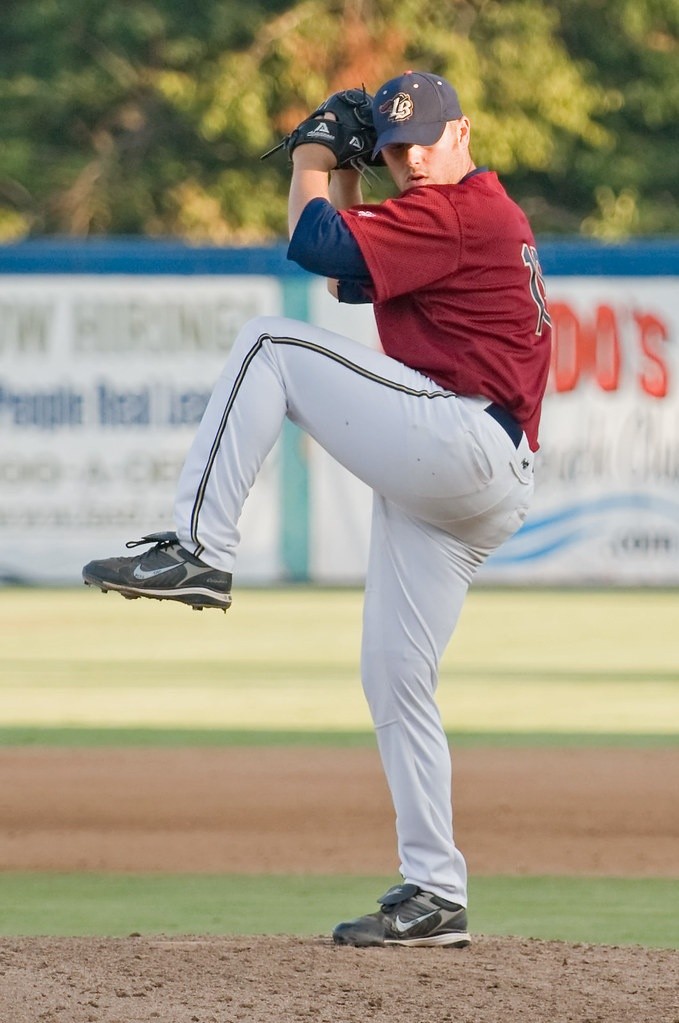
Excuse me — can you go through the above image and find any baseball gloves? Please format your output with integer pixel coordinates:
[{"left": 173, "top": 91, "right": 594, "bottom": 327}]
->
[{"left": 282, "top": 87, "right": 387, "bottom": 169}]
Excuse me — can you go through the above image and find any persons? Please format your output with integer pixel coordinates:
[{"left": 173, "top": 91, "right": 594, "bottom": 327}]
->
[{"left": 82, "top": 69, "right": 554, "bottom": 947}]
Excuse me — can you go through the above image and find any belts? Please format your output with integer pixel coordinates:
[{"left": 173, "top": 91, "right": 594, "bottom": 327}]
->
[{"left": 484, "top": 402, "right": 523, "bottom": 451}]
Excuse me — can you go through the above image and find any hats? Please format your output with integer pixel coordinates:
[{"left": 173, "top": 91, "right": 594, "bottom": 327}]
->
[{"left": 371, "top": 70, "right": 462, "bottom": 161}]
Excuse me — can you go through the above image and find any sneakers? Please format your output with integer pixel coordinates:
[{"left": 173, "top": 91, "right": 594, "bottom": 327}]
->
[
  {"left": 81, "top": 529, "right": 233, "bottom": 610},
  {"left": 333, "top": 883, "right": 471, "bottom": 948}
]
